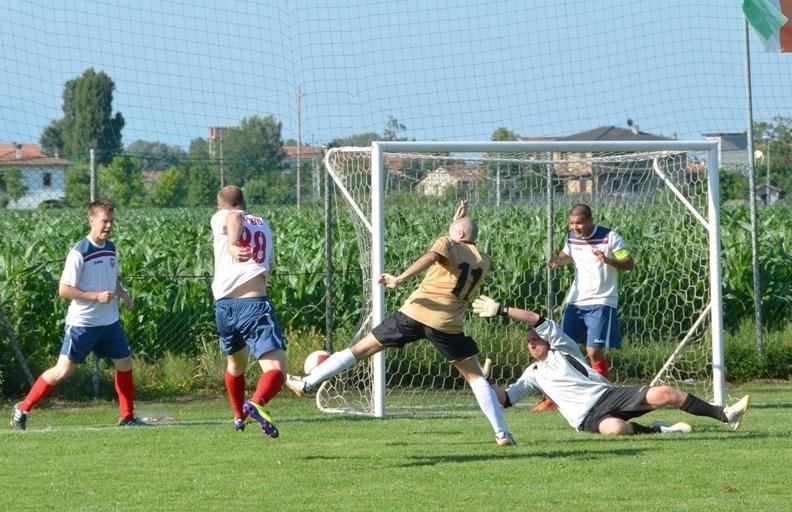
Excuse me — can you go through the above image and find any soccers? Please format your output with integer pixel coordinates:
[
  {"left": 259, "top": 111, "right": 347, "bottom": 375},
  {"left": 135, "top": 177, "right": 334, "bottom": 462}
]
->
[{"left": 305, "top": 350, "right": 334, "bottom": 381}]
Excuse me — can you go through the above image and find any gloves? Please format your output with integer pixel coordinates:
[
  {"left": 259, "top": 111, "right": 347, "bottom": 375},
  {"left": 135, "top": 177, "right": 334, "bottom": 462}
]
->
[{"left": 471, "top": 294, "right": 508, "bottom": 318}]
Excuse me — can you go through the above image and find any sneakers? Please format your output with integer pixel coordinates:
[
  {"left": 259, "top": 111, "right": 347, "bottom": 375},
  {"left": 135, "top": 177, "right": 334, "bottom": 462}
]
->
[
  {"left": 117, "top": 417, "right": 145, "bottom": 427},
  {"left": 652, "top": 421, "right": 692, "bottom": 433},
  {"left": 723, "top": 396, "right": 749, "bottom": 430},
  {"left": 12, "top": 402, "right": 28, "bottom": 430},
  {"left": 495, "top": 432, "right": 516, "bottom": 445},
  {"left": 236, "top": 401, "right": 278, "bottom": 438},
  {"left": 285, "top": 372, "right": 311, "bottom": 398}
]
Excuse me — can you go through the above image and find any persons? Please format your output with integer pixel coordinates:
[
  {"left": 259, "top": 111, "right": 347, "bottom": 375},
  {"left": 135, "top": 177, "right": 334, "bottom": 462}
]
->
[
  {"left": 287, "top": 198, "right": 516, "bottom": 446},
  {"left": 469, "top": 291, "right": 751, "bottom": 437},
  {"left": 10, "top": 200, "right": 154, "bottom": 431},
  {"left": 531, "top": 201, "right": 636, "bottom": 411},
  {"left": 210, "top": 184, "right": 287, "bottom": 439}
]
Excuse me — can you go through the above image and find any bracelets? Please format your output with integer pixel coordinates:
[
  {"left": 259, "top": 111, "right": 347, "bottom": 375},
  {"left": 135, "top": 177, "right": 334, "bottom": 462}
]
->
[{"left": 500, "top": 302, "right": 509, "bottom": 317}]
